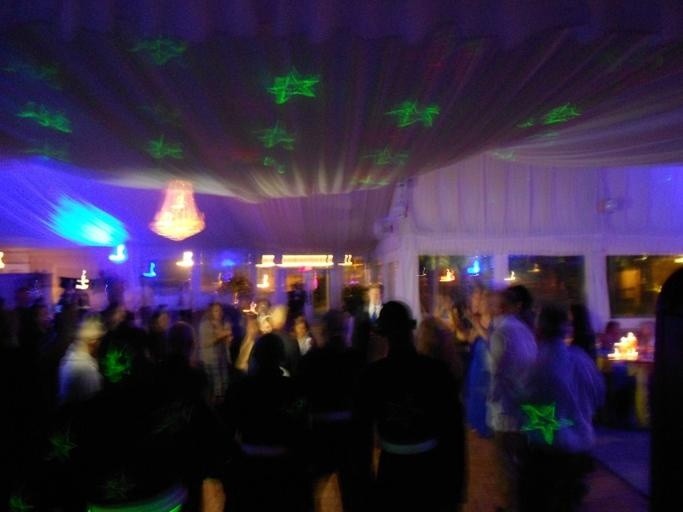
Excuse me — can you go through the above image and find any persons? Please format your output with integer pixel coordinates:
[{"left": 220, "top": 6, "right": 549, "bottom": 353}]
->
[{"left": 0, "top": 258, "right": 683, "bottom": 512}]
